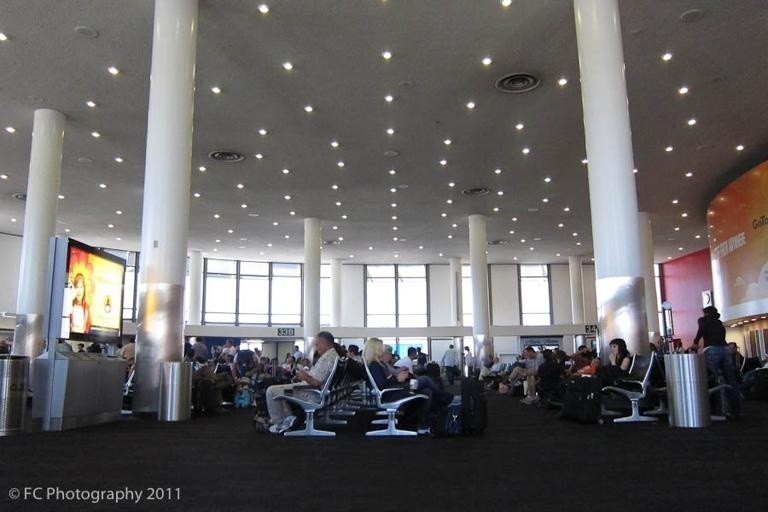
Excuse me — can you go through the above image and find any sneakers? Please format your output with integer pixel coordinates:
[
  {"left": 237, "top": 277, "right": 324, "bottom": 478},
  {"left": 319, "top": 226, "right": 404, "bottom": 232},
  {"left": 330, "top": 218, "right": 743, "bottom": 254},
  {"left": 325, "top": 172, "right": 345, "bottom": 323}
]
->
[
  {"left": 447, "top": 396, "right": 462, "bottom": 408},
  {"left": 279, "top": 415, "right": 299, "bottom": 434},
  {"left": 418, "top": 426, "right": 431, "bottom": 434},
  {"left": 520, "top": 397, "right": 538, "bottom": 405},
  {"left": 268, "top": 424, "right": 283, "bottom": 434}
]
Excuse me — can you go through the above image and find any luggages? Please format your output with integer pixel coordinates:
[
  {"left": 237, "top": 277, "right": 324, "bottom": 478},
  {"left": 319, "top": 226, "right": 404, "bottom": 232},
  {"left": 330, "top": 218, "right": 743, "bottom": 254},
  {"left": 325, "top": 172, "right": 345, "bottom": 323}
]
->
[
  {"left": 427, "top": 361, "right": 440, "bottom": 377},
  {"left": 461, "top": 377, "right": 487, "bottom": 432},
  {"left": 564, "top": 374, "right": 600, "bottom": 424}
]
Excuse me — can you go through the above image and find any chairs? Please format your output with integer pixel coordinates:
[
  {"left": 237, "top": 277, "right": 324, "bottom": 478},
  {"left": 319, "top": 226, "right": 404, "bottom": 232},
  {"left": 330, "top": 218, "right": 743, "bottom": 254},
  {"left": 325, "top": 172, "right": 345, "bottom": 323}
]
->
[
  {"left": 192, "top": 352, "right": 429, "bottom": 436},
  {"left": 121, "top": 369, "right": 136, "bottom": 415},
  {"left": 503, "top": 350, "right": 768, "bottom": 423}
]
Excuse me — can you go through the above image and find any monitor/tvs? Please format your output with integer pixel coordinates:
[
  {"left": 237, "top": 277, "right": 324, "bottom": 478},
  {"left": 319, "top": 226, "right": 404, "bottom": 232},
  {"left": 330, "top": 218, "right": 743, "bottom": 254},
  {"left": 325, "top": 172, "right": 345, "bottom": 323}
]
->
[{"left": 58, "top": 235, "right": 127, "bottom": 343}]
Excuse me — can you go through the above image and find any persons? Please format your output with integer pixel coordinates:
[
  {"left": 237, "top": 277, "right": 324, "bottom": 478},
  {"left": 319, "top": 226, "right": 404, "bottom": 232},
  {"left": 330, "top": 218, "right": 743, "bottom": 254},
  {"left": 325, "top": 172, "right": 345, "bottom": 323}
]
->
[
  {"left": 69, "top": 273, "right": 91, "bottom": 334},
  {"left": 76, "top": 304, "right": 768, "bottom": 436}
]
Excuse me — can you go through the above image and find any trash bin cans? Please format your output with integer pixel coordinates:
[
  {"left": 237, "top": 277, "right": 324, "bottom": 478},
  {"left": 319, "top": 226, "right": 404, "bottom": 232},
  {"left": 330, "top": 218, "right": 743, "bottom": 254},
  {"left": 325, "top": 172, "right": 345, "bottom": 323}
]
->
[
  {"left": 0, "top": 355, "right": 30, "bottom": 436},
  {"left": 664, "top": 354, "right": 709, "bottom": 427},
  {"left": 158, "top": 362, "right": 193, "bottom": 421}
]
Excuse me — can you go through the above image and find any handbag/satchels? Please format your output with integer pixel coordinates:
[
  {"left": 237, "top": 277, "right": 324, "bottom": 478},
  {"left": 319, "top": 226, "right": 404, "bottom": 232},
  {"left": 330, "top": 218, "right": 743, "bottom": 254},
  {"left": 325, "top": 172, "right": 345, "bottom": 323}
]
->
[
  {"left": 453, "top": 367, "right": 461, "bottom": 377},
  {"left": 446, "top": 408, "right": 463, "bottom": 435},
  {"left": 427, "top": 411, "right": 444, "bottom": 438},
  {"left": 514, "top": 386, "right": 523, "bottom": 397}
]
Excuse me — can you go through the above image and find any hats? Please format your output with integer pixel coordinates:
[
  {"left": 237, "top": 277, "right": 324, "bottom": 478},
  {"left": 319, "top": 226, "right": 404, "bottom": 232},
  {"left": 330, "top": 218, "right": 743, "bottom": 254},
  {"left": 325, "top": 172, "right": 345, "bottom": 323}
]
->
[{"left": 349, "top": 345, "right": 358, "bottom": 352}]
